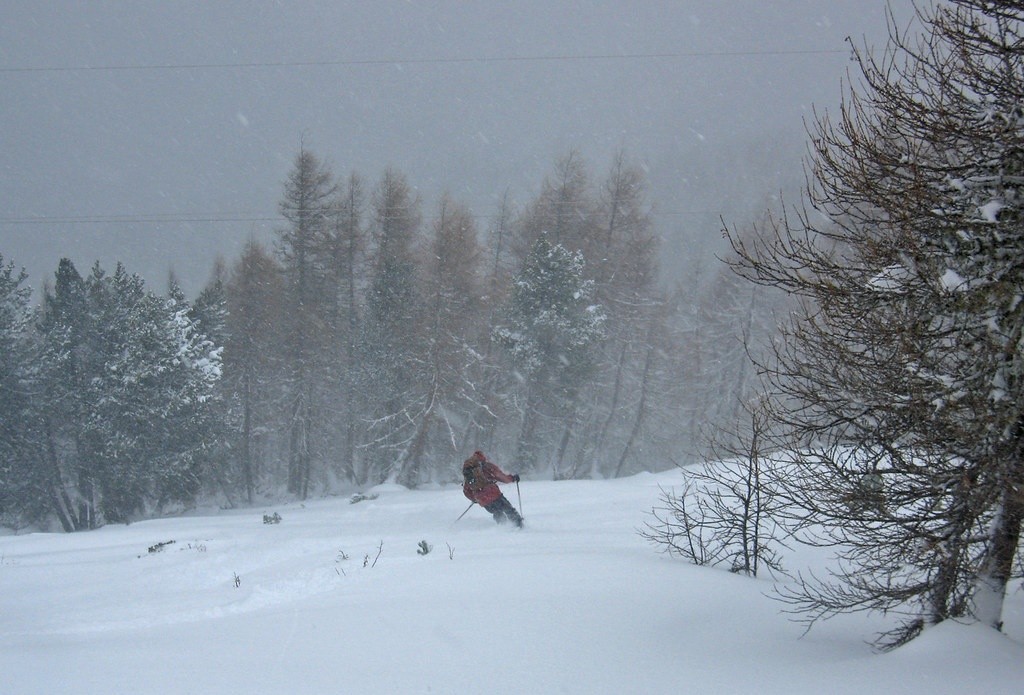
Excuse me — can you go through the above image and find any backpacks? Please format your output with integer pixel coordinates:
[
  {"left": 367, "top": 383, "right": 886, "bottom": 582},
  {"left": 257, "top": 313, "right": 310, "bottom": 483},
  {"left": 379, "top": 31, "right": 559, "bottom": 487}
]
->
[{"left": 462, "top": 457, "right": 495, "bottom": 491}]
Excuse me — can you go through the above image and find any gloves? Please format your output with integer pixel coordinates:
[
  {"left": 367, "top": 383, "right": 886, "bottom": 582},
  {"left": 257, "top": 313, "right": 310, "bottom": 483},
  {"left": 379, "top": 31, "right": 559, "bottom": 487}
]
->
[{"left": 512, "top": 474, "right": 520, "bottom": 483}]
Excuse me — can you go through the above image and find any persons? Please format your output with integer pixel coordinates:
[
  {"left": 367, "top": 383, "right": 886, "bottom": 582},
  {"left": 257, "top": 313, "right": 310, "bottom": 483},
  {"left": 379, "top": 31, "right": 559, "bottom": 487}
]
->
[{"left": 462, "top": 450, "right": 524, "bottom": 529}]
